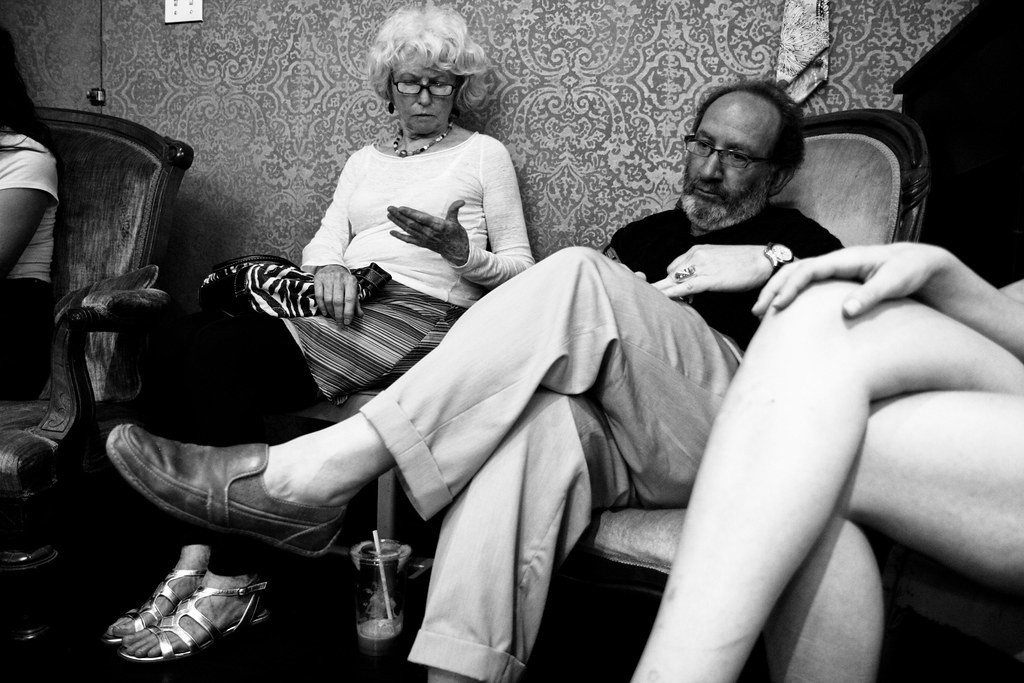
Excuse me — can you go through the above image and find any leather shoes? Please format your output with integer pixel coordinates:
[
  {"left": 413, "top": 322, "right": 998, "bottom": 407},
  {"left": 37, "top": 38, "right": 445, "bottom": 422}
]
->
[{"left": 105, "top": 423, "right": 348, "bottom": 554}]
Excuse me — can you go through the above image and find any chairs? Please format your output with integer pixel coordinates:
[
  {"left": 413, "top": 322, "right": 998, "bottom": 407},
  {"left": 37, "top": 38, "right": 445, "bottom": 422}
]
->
[
  {"left": 295, "top": 388, "right": 434, "bottom": 578},
  {"left": 573, "top": 110, "right": 931, "bottom": 597},
  {"left": 0, "top": 107, "right": 195, "bottom": 642}
]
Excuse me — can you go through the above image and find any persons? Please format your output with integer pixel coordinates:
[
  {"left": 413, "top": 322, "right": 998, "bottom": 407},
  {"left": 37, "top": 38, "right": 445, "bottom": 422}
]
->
[
  {"left": 633, "top": 242, "right": 1024, "bottom": 683},
  {"left": 104, "top": 7, "right": 846, "bottom": 683},
  {"left": 0, "top": 26, "right": 58, "bottom": 400}
]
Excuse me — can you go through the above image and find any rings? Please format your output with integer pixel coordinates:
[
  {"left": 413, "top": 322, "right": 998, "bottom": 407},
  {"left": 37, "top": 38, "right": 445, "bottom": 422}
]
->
[{"left": 673, "top": 266, "right": 698, "bottom": 283}]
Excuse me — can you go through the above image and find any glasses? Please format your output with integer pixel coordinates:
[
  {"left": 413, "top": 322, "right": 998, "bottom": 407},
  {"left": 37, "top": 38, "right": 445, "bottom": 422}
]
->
[
  {"left": 390, "top": 67, "right": 462, "bottom": 96},
  {"left": 684, "top": 135, "right": 775, "bottom": 169}
]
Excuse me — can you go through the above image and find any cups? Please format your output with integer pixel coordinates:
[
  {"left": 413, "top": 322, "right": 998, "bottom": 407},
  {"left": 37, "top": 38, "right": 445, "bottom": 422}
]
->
[{"left": 357, "top": 544, "right": 403, "bottom": 641}]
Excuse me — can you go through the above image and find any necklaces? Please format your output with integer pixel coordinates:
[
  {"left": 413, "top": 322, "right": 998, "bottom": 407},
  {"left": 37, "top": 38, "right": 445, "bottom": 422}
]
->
[{"left": 393, "top": 118, "right": 453, "bottom": 156}]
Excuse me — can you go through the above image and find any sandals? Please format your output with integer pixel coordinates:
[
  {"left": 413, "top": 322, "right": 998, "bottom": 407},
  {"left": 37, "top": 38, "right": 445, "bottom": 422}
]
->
[
  {"left": 117, "top": 580, "right": 270, "bottom": 662},
  {"left": 100, "top": 567, "right": 206, "bottom": 644}
]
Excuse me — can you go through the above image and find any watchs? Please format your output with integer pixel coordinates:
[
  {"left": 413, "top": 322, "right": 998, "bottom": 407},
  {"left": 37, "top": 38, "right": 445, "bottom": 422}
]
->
[{"left": 765, "top": 243, "right": 794, "bottom": 287}]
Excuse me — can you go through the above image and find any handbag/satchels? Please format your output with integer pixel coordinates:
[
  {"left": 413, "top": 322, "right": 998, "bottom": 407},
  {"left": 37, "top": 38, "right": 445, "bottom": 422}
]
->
[{"left": 198, "top": 254, "right": 392, "bottom": 319}]
